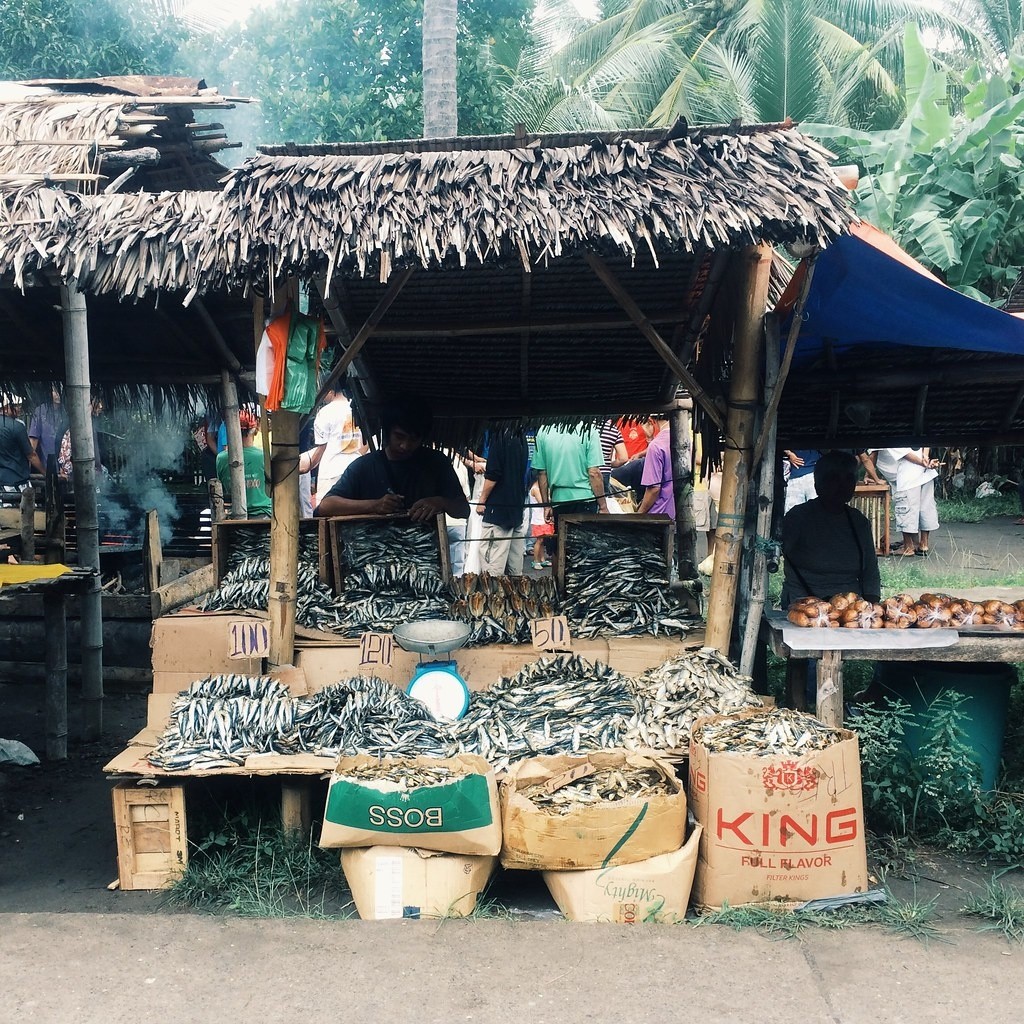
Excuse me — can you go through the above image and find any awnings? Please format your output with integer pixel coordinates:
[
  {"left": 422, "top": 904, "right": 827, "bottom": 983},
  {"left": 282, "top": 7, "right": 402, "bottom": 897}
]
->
[{"left": 764, "top": 219, "right": 1024, "bottom": 453}]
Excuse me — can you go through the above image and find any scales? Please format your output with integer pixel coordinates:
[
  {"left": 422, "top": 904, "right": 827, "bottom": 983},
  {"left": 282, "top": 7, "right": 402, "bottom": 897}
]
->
[{"left": 393, "top": 619, "right": 473, "bottom": 725}]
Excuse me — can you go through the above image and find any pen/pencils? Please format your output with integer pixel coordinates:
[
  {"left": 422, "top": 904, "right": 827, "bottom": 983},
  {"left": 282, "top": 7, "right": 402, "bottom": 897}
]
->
[{"left": 388, "top": 488, "right": 396, "bottom": 495}]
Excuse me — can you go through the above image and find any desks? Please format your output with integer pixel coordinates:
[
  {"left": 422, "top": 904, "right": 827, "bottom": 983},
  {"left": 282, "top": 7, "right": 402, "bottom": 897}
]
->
[{"left": 767, "top": 609, "right": 1024, "bottom": 729}]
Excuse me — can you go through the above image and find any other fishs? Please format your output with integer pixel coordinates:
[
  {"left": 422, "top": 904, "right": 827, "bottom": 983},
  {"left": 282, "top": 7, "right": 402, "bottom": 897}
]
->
[
  {"left": 563, "top": 523, "right": 704, "bottom": 642},
  {"left": 199, "top": 522, "right": 448, "bottom": 636},
  {"left": 450, "top": 572, "right": 559, "bottom": 648},
  {"left": 674, "top": 645, "right": 841, "bottom": 756},
  {"left": 294, "top": 674, "right": 459, "bottom": 787},
  {"left": 139, "top": 673, "right": 295, "bottom": 770},
  {"left": 456, "top": 653, "right": 681, "bottom": 810}
]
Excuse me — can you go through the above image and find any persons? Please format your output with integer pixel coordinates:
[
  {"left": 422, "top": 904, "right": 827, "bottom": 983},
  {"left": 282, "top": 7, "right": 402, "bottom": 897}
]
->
[
  {"left": 783, "top": 450, "right": 818, "bottom": 516},
  {"left": 690, "top": 426, "right": 725, "bottom": 557},
  {"left": 299, "top": 369, "right": 382, "bottom": 518},
  {"left": 0, "top": 380, "right": 109, "bottom": 548},
  {"left": 313, "top": 423, "right": 470, "bottom": 523},
  {"left": 830, "top": 447, "right": 946, "bottom": 557},
  {"left": 779, "top": 450, "right": 881, "bottom": 611},
  {"left": 207, "top": 401, "right": 272, "bottom": 521},
  {"left": 423, "top": 412, "right": 679, "bottom": 576}
]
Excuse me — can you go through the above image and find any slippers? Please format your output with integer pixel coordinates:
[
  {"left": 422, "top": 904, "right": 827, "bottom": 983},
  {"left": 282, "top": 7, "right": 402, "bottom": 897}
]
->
[
  {"left": 541, "top": 560, "right": 552, "bottom": 567},
  {"left": 532, "top": 562, "right": 543, "bottom": 570},
  {"left": 892, "top": 548, "right": 915, "bottom": 557},
  {"left": 889, "top": 539, "right": 905, "bottom": 548},
  {"left": 915, "top": 545, "right": 929, "bottom": 557}
]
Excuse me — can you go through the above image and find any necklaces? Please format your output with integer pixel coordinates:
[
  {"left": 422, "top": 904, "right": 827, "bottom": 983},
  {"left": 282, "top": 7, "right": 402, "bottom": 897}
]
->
[{"left": 658, "top": 420, "right": 669, "bottom": 430}]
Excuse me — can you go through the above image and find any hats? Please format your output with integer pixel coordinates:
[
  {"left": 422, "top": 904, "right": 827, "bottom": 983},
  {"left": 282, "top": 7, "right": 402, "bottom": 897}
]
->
[
  {"left": 0, "top": 392, "right": 26, "bottom": 407},
  {"left": 239, "top": 410, "right": 256, "bottom": 429}
]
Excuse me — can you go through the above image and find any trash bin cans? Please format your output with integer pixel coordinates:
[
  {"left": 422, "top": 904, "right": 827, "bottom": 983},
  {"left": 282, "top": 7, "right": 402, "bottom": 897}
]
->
[{"left": 875, "top": 662, "right": 1019, "bottom": 796}]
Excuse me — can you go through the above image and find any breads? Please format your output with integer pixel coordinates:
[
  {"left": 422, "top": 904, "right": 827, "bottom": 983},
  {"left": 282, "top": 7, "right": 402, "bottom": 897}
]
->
[{"left": 786, "top": 593, "right": 1024, "bottom": 630}]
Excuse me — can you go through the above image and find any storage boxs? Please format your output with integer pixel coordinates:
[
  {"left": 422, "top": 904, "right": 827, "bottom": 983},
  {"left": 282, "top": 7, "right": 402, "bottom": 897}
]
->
[
  {"left": 557, "top": 512, "right": 673, "bottom": 596},
  {"left": 327, "top": 511, "right": 451, "bottom": 595},
  {"left": 339, "top": 846, "right": 496, "bottom": 919},
  {"left": 212, "top": 516, "right": 332, "bottom": 597},
  {"left": 318, "top": 754, "right": 502, "bottom": 857},
  {"left": 152, "top": 672, "right": 261, "bottom": 693},
  {"left": 292, "top": 626, "right": 706, "bottom": 703},
  {"left": 110, "top": 775, "right": 190, "bottom": 892},
  {"left": 148, "top": 614, "right": 262, "bottom": 673},
  {"left": 542, "top": 808, "right": 702, "bottom": 921},
  {"left": 687, "top": 710, "right": 868, "bottom": 910},
  {"left": 497, "top": 748, "right": 686, "bottom": 870}
]
